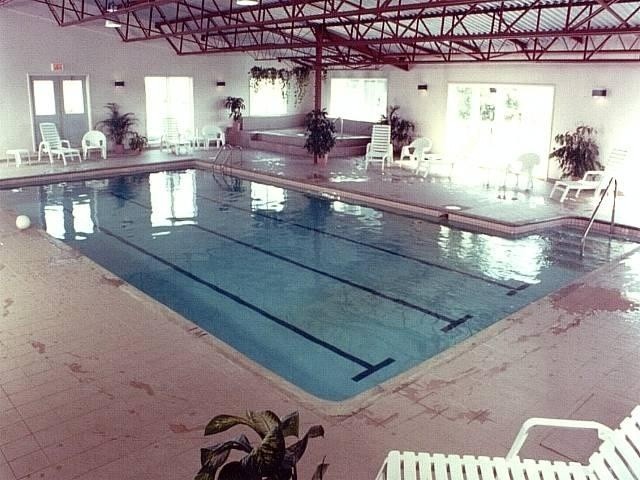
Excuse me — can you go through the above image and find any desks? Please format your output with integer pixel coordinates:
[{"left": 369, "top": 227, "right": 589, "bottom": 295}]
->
[{"left": 6, "top": 150, "right": 31, "bottom": 168}]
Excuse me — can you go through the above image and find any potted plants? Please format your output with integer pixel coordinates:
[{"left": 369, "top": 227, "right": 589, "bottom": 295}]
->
[
  {"left": 302, "top": 107, "right": 339, "bottom": 165},
  {"left": 225, "top": 96, "right": 245, "bottom": 134},
  {"left": 93, "top": 102, "right": 149, "bottom": 155},
  {"left": 248, "top": 64, "right": 312, "bottom": 108},
  {"left": 548, "top": 126, "right": 606, "bottom": 181}
]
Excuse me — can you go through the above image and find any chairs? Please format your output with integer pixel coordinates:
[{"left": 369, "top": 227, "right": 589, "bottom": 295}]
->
[
  {"left": 503, "top": 153, "right": 541, "bottom": 189},
  {"left": 365, "top": 124, "right": 455, "bottom": 179},
  {"left": 550, "top": 147, "right": 629, "bottom": 203},
  {"left": 37, "top": 122, "right": 107, "bottom": 166},
  {"left": 372, "top": 402, "right": 640, "bottom": 480},
  {"left": 160, "top": 116, "right": 226, "bottom": 156}
]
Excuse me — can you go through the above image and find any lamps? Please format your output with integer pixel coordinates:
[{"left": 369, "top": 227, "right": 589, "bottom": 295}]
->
[{"left": 104, "top": 2, "right": 123, "bottom": 28}]
